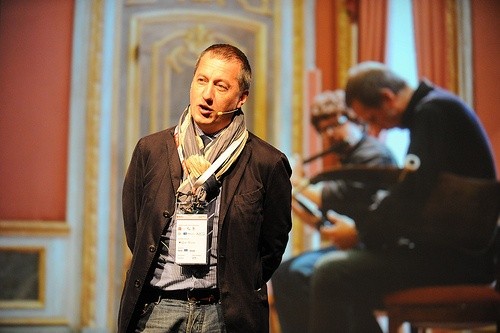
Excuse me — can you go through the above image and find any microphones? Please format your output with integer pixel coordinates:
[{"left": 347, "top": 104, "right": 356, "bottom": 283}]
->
[{"left": 218, "top": 109, "right": 238, "bottom": 115}]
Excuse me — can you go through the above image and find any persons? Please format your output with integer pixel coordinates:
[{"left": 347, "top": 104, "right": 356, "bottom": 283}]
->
[
  {"left": 118, "top": 44, "right": 292, "bottom": 333},
  {"left": 272, "top": 61, "right": 500, "bottom": 333}
]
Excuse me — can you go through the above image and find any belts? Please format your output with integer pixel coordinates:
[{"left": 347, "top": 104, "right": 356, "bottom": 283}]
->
[{"left": 151, "top": 287, "right": 219, "bottom": 304}]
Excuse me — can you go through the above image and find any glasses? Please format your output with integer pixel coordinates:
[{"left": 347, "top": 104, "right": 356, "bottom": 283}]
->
[{"left": 316, "top": 115, "right": 350, "bottom": 136}]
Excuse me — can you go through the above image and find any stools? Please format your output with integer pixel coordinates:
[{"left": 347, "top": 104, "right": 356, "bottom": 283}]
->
[{"left": 382, "top": 287, "right": 500, "bottom": 333}]
